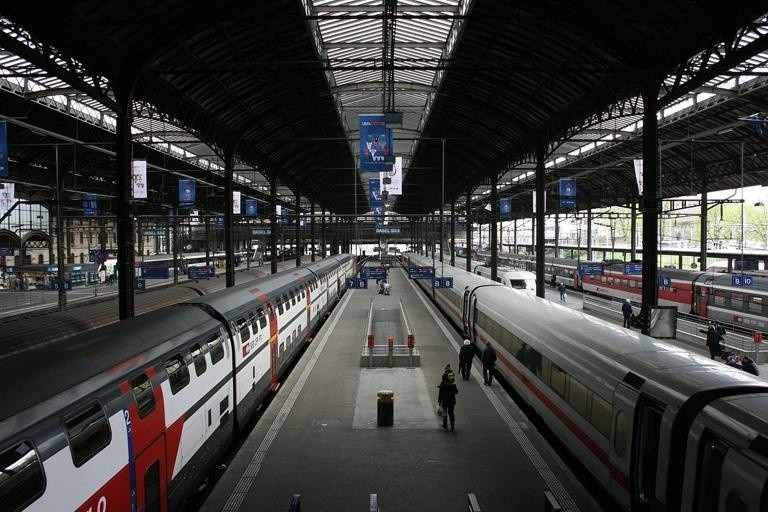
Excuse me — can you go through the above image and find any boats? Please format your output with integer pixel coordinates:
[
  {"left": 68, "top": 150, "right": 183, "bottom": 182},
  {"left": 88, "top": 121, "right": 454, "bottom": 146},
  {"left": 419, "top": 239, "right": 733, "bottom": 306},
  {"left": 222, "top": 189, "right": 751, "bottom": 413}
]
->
[{"left": 0, "top": 253, "right": 359, "bottom": 511}]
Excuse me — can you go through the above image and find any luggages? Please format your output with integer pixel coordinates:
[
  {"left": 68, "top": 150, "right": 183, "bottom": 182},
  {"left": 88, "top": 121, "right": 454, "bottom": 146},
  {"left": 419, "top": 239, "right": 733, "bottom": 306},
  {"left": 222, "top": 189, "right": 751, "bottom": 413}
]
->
[{"left": 721, "top": 341, "right": 730, "bottom": 359}]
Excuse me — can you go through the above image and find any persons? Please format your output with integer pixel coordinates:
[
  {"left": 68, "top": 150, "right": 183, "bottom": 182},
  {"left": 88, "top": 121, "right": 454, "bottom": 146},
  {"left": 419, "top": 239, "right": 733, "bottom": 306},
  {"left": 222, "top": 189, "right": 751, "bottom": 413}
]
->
[
  {"left": 721, "top": 351, "right": 759, "bottom": 376},
  {"left": 620, "top": 297, "right": 633, "bottom": 329},
  {"left": 437, "top": 370, "right": 459, "bottom": 433},
  {"left": 457, "top": 338, "right": 476, "bottom": 381},
  {"left": 558, "top": 282, "right": 566, "bottom": 301},
  {"left": 514, "top": 341, "right": 544, "bottom": 378},
  {"left": 480, "top": 340, "right": 498, "bottom": 386},
  {"left": 711, "top": 320, "right": 726, "bottom": 356},
  {"left": 696, "top": 323, "right": 726, "bottom": 359}
]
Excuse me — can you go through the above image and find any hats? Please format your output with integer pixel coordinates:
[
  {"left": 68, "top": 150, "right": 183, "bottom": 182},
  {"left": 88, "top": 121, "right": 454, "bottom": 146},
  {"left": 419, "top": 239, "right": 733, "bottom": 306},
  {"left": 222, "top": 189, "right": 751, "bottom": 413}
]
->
[
  {"left": 448, "top": 373, "right": 455, "bottom": 381},
  {"left": 486, "top": 341, "right": 491, "bottom": 347},
  {"left": 464, "top": 339, "right": 471, "bottom": 345}
]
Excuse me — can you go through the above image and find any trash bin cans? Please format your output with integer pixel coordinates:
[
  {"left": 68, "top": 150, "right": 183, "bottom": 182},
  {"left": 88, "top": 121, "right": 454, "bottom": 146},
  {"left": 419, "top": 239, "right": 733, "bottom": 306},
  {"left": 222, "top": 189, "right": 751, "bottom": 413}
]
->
[{"left": 377, "top": 391, "right": 393, "bottom": 426}]
